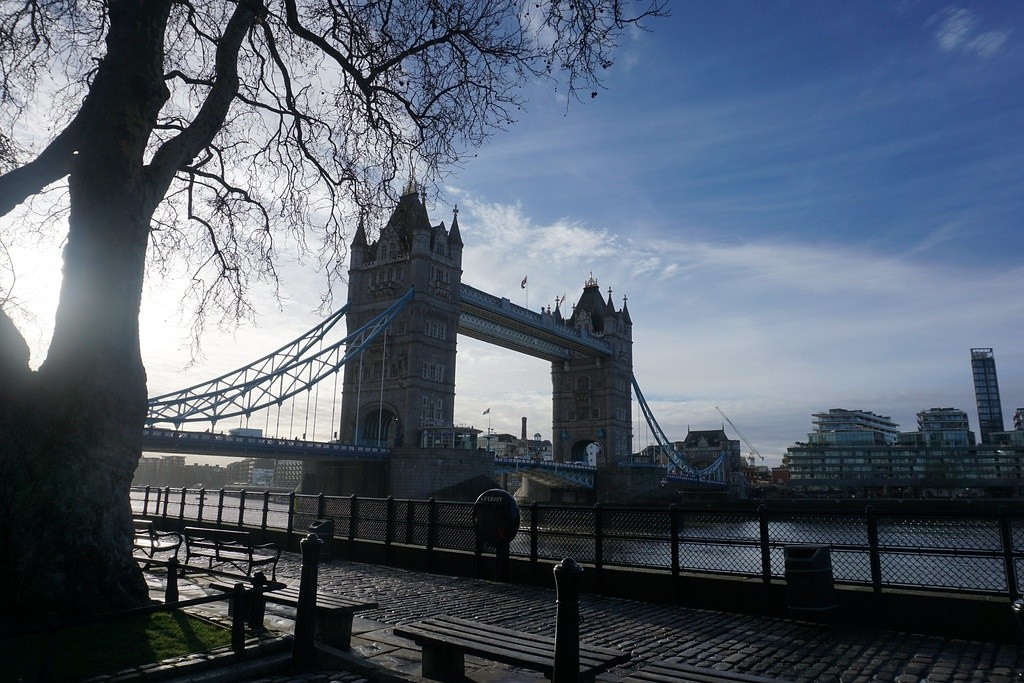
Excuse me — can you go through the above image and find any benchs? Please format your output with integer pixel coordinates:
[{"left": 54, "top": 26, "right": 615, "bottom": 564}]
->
[
  {"left": 131, "top": 517, "right": 182, "bottom": 574},
  {"left": 180, "top": 526, "right": 283, "bottom": 586},
  {"left": 395, "top": 613, "right": 631, "bottom": 683},
  {"left": 209, "top": 578, "right": 378, "bottom": 654},
  {"left": 619, "top": 659, "right": 793, "bottom": 683}
]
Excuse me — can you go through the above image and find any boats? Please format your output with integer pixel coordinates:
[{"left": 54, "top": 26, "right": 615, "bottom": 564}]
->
[{"left": 751, "top": 477, "right": 1024, "bottom": 517}]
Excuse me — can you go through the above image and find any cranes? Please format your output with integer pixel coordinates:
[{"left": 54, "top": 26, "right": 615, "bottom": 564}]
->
[{"left": 716, "top": 407, "right": 764, "bottom": 466}]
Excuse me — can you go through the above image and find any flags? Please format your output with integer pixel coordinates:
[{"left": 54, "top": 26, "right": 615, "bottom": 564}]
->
[
  {"left": 520, "top": 276, "right": 528, "bottom": 289},
  {"left": 482, "top": 407, "right": 490, "bottom": 415}
]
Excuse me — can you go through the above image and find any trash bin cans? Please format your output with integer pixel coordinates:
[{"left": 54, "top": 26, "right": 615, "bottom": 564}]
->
[
  {"left": 308, "top": 519, "right": 333, "bottom": 559},
  {"left": 785, "top": 544, "right": 837, "bottom": 624}
]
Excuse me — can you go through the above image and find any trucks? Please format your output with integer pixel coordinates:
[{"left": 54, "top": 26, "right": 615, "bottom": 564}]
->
[{"left": 229, "top": 428, "right": 262, "bottom": 444}]
